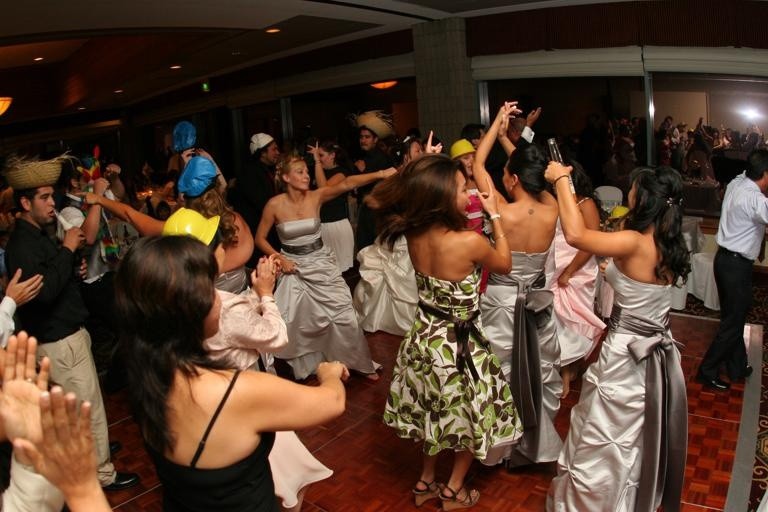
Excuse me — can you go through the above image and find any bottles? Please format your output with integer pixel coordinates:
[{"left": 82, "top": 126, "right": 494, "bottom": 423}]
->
[
  {"left": 53, "top": 210, "right": 88, "bottom": 249},
  {"left": 547, "top": 137, "right": 578, "bottom": 203}
]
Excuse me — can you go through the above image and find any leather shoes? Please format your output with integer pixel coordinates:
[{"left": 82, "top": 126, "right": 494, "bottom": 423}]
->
[
  {"left": 110, "top": 441, "right": 121, "bottom": 453},
  {"left": 708, "top": 378, "right": 731, "bottom": 390},
  {"left": 739, "top": 364, "right": 752, "bottom": 377},
  {"left": 102, "top": 473, "right": 139, "bottom": 491}
]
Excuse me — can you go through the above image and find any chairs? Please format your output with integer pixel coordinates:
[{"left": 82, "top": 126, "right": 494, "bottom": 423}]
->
[
  {"left": 665, "top": 226, "right": 695, "bottom": 312},
  {"left": 692, "top": 250, "right": 721, "bottom": 310}
]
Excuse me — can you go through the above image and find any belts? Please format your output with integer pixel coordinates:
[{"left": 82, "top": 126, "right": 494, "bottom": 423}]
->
[{"left": 718, "top": 246, "right": 754, "bottom": 263}]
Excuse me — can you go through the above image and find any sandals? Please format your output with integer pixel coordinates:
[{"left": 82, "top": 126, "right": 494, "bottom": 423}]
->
[
  {"left": 439, "top": 484, "right": 480, "bottom": 511},
  {"left": 412, "top": 479, "right": 442, "bottom": 507}
]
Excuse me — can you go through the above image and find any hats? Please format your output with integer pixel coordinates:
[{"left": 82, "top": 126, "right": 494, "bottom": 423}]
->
[
  {"left": 350, "top": 109, "right": 396, "bottom": 139},
  {"left": 249, "top": 132, "right": 275, "bottom": 154},
  {"left": 177, "top": 156, "right": 221, "bottom": 199},
  {"left": 171, "top": 121, "right": 197, "bottom": 152}
]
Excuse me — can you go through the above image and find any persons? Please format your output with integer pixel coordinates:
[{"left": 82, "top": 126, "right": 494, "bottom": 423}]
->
[
  {"left": 599, "top": 99, "right": 768, "bottom": 389},
  {"left": 236, "top": 100, "right": 688, "bottom": 512},
  {"left": 0, "top": 120, "right": 352, "bottom": 512}
]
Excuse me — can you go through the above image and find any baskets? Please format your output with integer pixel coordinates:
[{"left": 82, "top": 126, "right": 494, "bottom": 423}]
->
[{"left": 5, "top": 159, "right": 64, "bottom": 190}]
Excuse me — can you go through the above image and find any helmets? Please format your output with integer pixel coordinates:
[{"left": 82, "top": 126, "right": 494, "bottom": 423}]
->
[
  {"left": 448, "top": 138, "right": 476, "bottom": 161},
  {"left": 161, "top": 207, "right": 221, "bottom": 246}
]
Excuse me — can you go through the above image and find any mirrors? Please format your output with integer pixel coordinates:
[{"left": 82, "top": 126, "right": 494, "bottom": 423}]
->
[
  {"left": 482, "top": 48, "right": 644, "bottom": 206},
  {"left": 643, "top": 47, "right": 766, "bottom": 217},
  {"left": 62, "top": 48, "right": 285, "bottom": 168},
  {"left": 285, "top": 49, "right": 420, "bottom": 151}
]
